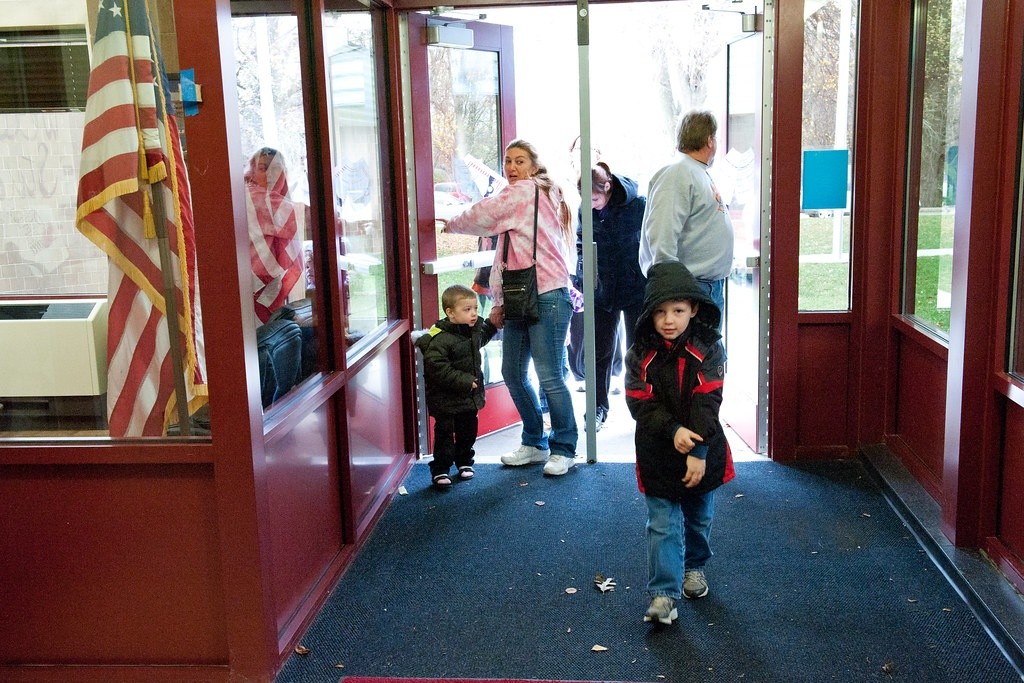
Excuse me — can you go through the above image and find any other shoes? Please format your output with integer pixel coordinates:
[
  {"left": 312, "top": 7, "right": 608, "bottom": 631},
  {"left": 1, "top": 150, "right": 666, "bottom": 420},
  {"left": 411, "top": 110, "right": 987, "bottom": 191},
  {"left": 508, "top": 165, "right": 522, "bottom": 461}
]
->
[{"left": 584, "top": 406, "right": 606, "bottom": 432}]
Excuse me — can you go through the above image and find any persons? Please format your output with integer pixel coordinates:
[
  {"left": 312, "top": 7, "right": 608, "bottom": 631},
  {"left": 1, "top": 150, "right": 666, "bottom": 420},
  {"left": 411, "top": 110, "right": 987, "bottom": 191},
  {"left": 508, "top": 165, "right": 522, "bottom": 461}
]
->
[
  {"left": 624, "top": 259, "right": 736, "bottom": 626},
  {"left": 424, "top": 284, "right": 504, "bottom": 489},
  {"left": 244, "top": 148, "right": 346, "bottom": 383},
  {"left": 576, "top": 161, "right": 647, "bottom": 433},
  {"left": 472, "top": 234, "right": 497, "bottom": 319},
  {"left": 438, "top": 138, "right": 580, "bottom": 476},
  {"left": 566, "top": 258, "right": 624, "bottom": 396},
  {"left": 638, "top": 111, "right": 735, "bottom": 361}
]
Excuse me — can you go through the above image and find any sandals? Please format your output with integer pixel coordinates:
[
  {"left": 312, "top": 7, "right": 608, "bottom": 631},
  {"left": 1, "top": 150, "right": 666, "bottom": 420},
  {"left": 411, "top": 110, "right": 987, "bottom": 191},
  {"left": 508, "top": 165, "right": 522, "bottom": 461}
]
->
[
  {"left": 431, "top": 473, "right": 453, "bottom": 487},
  {"left": 458, "top": 465, "right": 475, "bottom": 479}
]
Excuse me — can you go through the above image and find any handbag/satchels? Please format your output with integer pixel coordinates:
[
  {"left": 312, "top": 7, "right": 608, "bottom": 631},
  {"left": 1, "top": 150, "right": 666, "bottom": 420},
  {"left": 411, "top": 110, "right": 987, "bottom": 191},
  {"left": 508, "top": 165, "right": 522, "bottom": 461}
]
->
[
  {"left": 502, "top": 259, "right": 540, "bottom": 322},
  {"left": 575, "top": 259, "right": 619, "bottom": 312}
]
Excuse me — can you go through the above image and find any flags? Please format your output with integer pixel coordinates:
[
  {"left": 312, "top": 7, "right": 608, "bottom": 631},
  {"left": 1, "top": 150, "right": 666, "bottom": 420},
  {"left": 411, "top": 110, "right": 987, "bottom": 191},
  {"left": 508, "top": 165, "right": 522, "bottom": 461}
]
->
[{"left": 74, "top": 0, "right": 209, "bottom": 442}]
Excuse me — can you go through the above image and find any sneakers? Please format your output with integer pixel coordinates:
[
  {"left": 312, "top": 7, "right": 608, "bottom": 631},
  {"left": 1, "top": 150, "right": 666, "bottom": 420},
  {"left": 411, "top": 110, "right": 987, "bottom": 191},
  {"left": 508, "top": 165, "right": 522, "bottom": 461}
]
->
[
  {"left": 577, "top": 379, "right": 588, "bottom": 392},
  {"left": 611, "top": 376, "right": 621, "bottom": 393},
  {"left": 500, "top": 444, "right": 551, "bottom": 465},
  {"left": 544, "top": 455, "right": 576, "bottom": 475},
  {"left": 643, "top": 597, "right": 679, "bottom": 625},
  {"left": 682, "top": 570, "right": 709, "bottom": 599}
]
